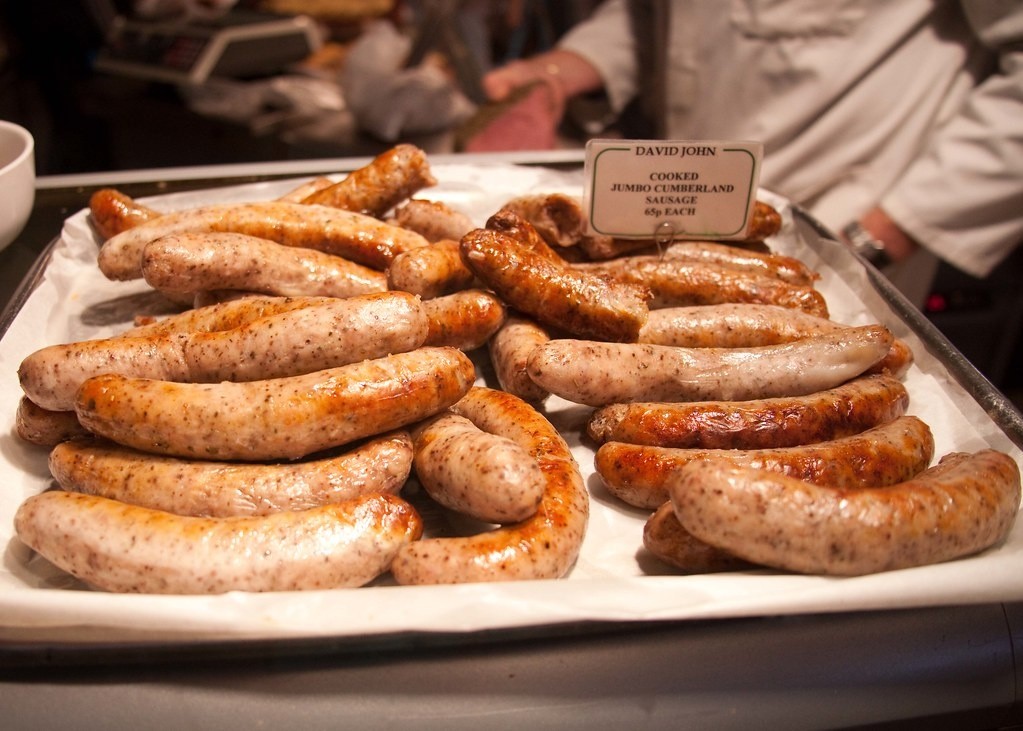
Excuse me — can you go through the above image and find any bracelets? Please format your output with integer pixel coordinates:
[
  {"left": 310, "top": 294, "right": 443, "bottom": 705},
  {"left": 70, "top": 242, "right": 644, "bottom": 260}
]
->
[
  {"left": 844, "top": 222, "right": 895, "bottom": 272},
  {"left": 525, "top": 59, "right": 567, "bottom": 124}
]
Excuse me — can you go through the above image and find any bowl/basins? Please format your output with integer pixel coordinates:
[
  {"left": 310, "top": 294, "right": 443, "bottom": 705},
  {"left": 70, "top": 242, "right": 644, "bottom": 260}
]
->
[{"left": 0, "top": 120, "right": 36, "bottom": 251}]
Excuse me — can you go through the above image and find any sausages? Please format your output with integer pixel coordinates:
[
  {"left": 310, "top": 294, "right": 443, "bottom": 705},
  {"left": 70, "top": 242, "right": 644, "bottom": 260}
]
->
[{"left": 13, "top": 144, "right": 1022, "bottom": 596}]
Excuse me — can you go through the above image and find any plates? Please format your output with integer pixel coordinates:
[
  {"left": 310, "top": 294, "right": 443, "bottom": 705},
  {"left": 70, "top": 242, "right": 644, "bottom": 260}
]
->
[{"left": 0, "top": 167, "right": 1021, "bottom": 648}]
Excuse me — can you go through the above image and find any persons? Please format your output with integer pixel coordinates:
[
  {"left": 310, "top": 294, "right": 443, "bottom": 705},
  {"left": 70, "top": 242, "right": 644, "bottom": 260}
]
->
[{"left": 483, "top": 0, "right": 1023, "bottom": 308}]
